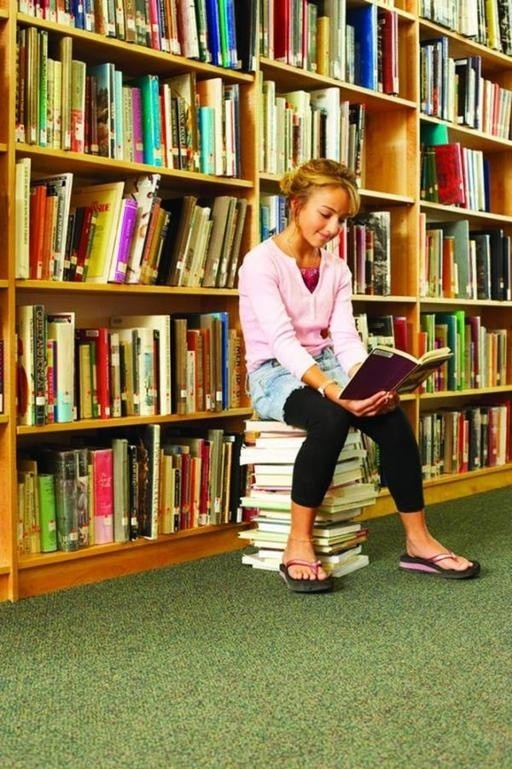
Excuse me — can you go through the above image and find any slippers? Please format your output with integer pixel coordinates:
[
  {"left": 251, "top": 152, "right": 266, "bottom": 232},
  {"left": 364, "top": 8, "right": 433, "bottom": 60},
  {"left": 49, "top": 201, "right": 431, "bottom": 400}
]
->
[
  {"left": 398, "top": 549, "right": 480, "bottom": 578},
  {"left": 279, "top": 559, "right": 332, "bottom": 591}
]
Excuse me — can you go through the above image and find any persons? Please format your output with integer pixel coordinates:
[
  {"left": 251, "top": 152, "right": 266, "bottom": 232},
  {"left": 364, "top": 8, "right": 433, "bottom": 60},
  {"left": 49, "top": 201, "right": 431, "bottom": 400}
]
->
[{"left": 237, "top": 161, "right": 479, "bottom": 592}]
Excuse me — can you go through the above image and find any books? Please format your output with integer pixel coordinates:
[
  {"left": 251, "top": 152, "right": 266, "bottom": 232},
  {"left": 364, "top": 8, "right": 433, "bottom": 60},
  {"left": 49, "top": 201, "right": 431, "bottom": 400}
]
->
[{"left": 0, "top": 0, "right": 512, "bottom": 579}]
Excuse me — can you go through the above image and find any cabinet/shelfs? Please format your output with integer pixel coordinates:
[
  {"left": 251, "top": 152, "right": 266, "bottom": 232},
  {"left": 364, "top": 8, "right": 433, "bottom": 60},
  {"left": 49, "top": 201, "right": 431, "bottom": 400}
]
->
[{"left": 2, "top": 0, "right": 510, "bottom": 604}]
